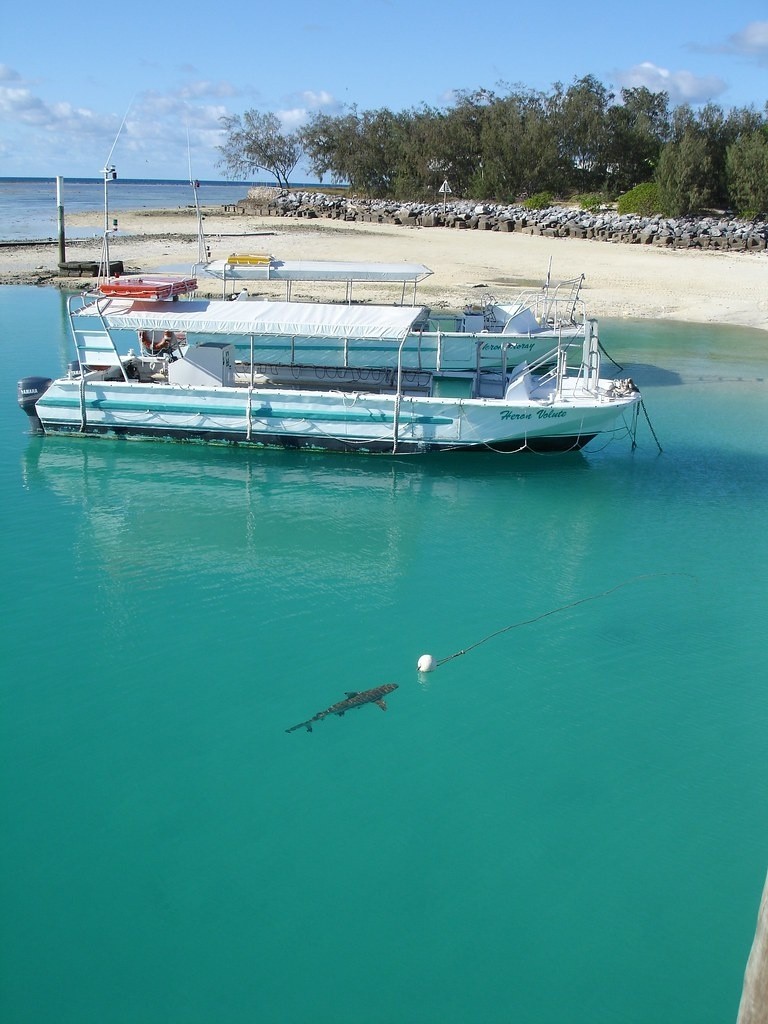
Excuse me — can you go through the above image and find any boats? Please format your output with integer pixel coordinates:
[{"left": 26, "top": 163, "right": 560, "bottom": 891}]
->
[{"left": 17, "top": 95, "right": 642, "bottom": 456}]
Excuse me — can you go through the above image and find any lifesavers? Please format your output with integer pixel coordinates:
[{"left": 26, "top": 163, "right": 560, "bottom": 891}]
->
[
  {"left": 139, "top": 331, "right": 172, "bottom": 350},
  {"left": 89, "top": 366, "right": 111, "bottom": 371}
]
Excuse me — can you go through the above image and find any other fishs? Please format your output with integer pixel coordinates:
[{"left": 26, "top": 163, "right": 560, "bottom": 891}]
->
[{"left": 284, "top": 683, "right": 400, "bottom": 734}]
